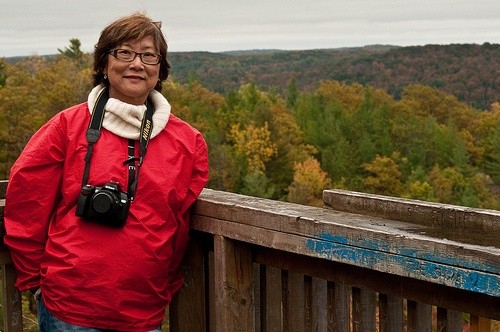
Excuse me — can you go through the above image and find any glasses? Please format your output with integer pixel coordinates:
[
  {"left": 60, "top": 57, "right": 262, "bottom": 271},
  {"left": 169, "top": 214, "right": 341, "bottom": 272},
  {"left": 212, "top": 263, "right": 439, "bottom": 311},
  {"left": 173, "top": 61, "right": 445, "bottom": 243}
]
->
[{"left": 100, "top": 49, "right": 162, "bottom": 65}]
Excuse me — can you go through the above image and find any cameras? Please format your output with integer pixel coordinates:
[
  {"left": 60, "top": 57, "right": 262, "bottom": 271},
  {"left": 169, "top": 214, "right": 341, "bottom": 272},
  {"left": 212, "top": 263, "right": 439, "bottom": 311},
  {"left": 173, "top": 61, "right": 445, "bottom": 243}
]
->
[{"left": 75, "top": 180, "right": 130, "bottom": 228}]
